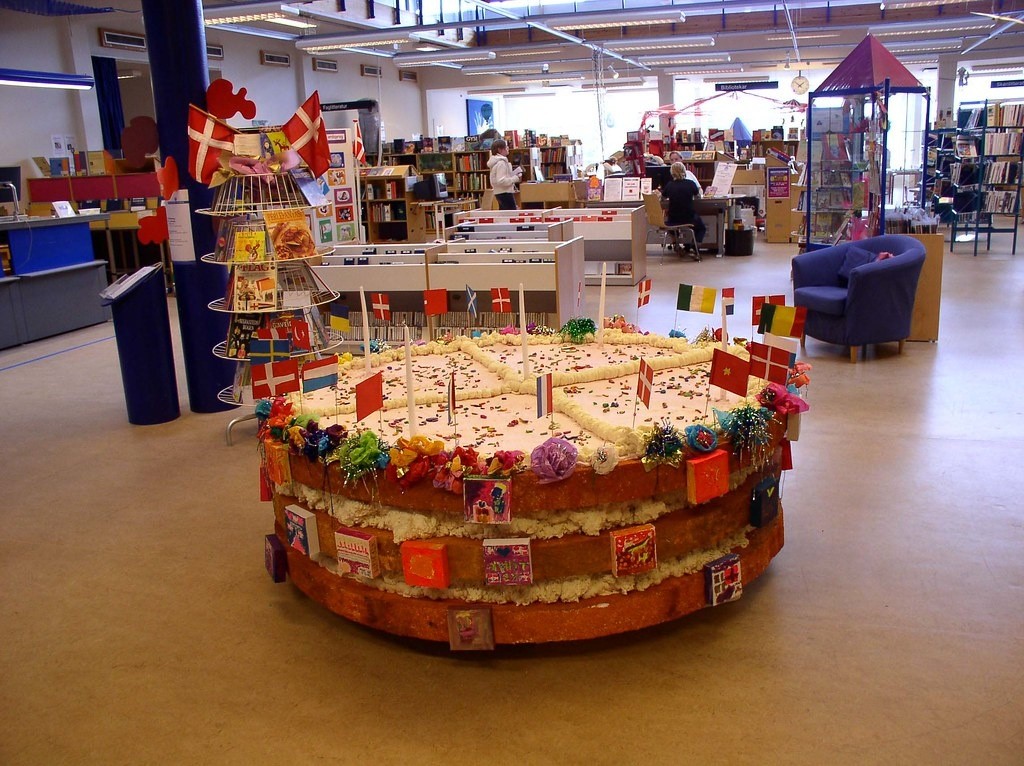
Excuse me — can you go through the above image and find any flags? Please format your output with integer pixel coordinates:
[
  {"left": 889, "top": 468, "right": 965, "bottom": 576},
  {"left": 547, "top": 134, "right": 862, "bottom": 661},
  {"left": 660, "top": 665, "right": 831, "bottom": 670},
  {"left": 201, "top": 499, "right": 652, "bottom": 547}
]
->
[
  {"left": 281, "top": 90, "right": 333, "bottom": 179},
  {"left": 637, "top": 277, "right": 734, "bottom": 316},
  {"left": 355, "top": 371, "right": 383, "bottom": 423},
  {"left": 637, "top": 358, "right": 654, "bottom": 410},
  {"left": 250, "top": 358, "right": 300, "bottom": 400},
  {"left": 423, "top": 289, "right": 448, "bottom": 316},
  {"left": 537, "top": 373, "right": 552, "bottom": 419},
  {"left": 490, "top": 287, "right": 511, "bottom": 313},
  {"left": 708, "top": 295, "right": 808, "bottom": 396},
  {"left": 371, "top": 293, "right": 392, "bottom": 321},
  {"left": 188, "top": 105, "right": 236, "bottom": 186},
  {"left": 448, "top": 372, "right": 456, "bottom": 427},
  {"left": 302, "top": 354, "right": 338, "bottom": 393},
  {"left": 352, "top": 118, "right": 368, "bottom": 166}
]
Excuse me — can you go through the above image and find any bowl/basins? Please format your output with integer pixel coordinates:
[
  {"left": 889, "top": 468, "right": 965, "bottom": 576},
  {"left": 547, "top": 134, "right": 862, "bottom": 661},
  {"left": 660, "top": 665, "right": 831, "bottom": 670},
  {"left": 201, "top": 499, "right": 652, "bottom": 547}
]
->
[{"left": 78, "top": 207, "right": 100, "bottom": 216}]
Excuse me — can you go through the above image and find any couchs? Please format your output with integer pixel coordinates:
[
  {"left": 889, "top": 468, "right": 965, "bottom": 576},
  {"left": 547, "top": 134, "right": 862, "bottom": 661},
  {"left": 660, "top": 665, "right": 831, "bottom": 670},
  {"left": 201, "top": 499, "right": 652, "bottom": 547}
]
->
[{"left": 790, "top": 232, "right": 927, "bottom": 364}]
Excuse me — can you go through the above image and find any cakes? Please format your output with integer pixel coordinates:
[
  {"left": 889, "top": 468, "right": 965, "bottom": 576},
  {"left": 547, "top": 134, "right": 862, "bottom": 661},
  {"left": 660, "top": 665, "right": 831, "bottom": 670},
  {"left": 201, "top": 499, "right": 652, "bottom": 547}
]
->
[{"left": 255, "top": 317, "right": 810, "bottom": 650}]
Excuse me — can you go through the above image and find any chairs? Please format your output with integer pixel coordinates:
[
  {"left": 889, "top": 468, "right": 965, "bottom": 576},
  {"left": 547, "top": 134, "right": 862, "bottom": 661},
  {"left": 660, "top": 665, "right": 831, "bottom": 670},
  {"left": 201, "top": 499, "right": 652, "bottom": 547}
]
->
[{"left": 643, "top": 193, "right": 702, "bottom": 267}]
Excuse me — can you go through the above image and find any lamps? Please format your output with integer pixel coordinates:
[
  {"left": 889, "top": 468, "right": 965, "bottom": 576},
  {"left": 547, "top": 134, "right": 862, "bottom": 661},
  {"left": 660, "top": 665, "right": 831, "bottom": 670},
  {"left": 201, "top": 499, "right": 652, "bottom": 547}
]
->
[
  {"left": 203, "top": 0, "right": 771, "bottom": 99},
  {"left": 972, "top": 61, "right": 1024, "bottom": 71},
  {"left": 896, "top": 54, "right": 940, "bottom": 64},
  {"left": 0, "top": 68, "right": 95, "bottom": 91},
  {"left": 784, "top": 53, "right": 791, "bottom": 70},
  {"left": 880, "top": 0, "right": 981, "bottom": 12},
  {"left": 882, "top": 39, "right": 964, "bottom": 52},
  {"left": 865, "top": 14, "right": 998, "bottom": 36}
]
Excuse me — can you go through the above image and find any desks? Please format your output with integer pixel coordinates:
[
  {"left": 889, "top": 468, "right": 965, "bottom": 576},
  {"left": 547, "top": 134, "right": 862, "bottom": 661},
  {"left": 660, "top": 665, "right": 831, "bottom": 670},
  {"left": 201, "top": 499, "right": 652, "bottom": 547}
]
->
[
  {"left": 586, "top": 199, "right": 727, "bottom": 258},
  {"left": 888, "top": 171, "right": 921, "bottom": 203},
  {"left": 0, "top": 212, "right": 118, "bottom": 350},
  {"left": 424, "top": 220, "right": 477, "bottom": 242}
]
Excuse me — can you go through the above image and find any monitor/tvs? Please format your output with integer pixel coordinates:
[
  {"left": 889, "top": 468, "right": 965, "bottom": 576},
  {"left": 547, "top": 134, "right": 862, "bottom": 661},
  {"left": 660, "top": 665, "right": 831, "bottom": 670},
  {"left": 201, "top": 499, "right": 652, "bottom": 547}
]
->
[
  {"left": 414, "top": 173, "right": 448, "bottom": 201},
  {"left": 645, "top": 166, "right": 673, "bottom": 192}
]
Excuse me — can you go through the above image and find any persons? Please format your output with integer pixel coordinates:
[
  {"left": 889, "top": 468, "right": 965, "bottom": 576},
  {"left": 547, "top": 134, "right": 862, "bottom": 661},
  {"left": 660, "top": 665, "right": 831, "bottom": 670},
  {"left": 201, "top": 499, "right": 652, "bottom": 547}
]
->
[
  {"left": 670, "top": 152, "right": 703, "bottom": 196},
  {"left": 487, "top": 140, "right": 522, "bottom": 210},
  {"left": 662, "top": 162, "right": 706, "bottom": 261}
]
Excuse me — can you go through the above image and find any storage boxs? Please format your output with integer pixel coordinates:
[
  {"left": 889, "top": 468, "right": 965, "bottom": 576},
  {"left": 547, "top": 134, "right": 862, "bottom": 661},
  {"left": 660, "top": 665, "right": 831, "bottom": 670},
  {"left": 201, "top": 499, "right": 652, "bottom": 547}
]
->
[
  {"left": 382, "top": 129, "right": 572, "bottom": 154},
  {"left": 627, "top": 128, "right": 800, "bottom": 141}
]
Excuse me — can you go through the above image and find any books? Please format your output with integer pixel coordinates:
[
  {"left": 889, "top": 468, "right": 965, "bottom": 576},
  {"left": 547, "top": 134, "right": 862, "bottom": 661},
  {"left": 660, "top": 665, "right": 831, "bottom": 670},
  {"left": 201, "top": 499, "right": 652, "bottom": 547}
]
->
[
  {"left": 927, "top": 104, "right": 1024, "bottom": 216},
  {"left": 367, "top": 129, "right": 575, "bottom": 210},
  {"left": 233, "top": 211, "right": 329, "bottom": 403}
]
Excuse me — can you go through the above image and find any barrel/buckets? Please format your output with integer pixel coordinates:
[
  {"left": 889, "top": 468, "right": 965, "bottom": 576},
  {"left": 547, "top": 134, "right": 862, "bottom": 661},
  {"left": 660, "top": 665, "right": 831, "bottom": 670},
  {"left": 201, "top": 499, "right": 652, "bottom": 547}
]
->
[{"left": 725, "top": 228, "right": 753, "bottom": 255}]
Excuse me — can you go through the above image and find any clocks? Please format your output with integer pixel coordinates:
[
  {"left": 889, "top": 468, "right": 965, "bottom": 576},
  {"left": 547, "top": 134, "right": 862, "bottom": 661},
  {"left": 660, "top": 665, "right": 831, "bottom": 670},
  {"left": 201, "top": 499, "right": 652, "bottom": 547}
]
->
[{"left": 790, "top": 76, "right": 810, "bottom": 96}]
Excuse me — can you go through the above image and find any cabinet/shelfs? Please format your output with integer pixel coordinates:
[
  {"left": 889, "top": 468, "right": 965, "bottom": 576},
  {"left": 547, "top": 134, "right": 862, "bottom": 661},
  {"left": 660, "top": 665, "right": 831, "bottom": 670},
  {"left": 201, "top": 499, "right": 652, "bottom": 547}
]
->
[
  {"left": 442, "top": 220, "right": 574, "bottom": 242},
  {"left": 452, "top": 208, "right": 647, "bottom": 286},
  {"left": 193, "top": 168, "right": 345, "bottom": 450},
  {"left": 540, "top": 146, "right": 574, "bottom": 180},
  {"left": 374, "top": 149, "right": 493, "bottom": 211},
  {"left": 508, "top": 145, "right": 540, "bottom": 189},
  {"left": 311, "top": 236, "right": 587, "bottom": 355},
  {"left": 359, "top": 164, "right": 426, "bottom": 244},
  {"left": 646, "top": 100, "right": 1024, "bottom": 342},
  {"left": 573, "top": 145, "right": 582, "bottom": 174}
]
